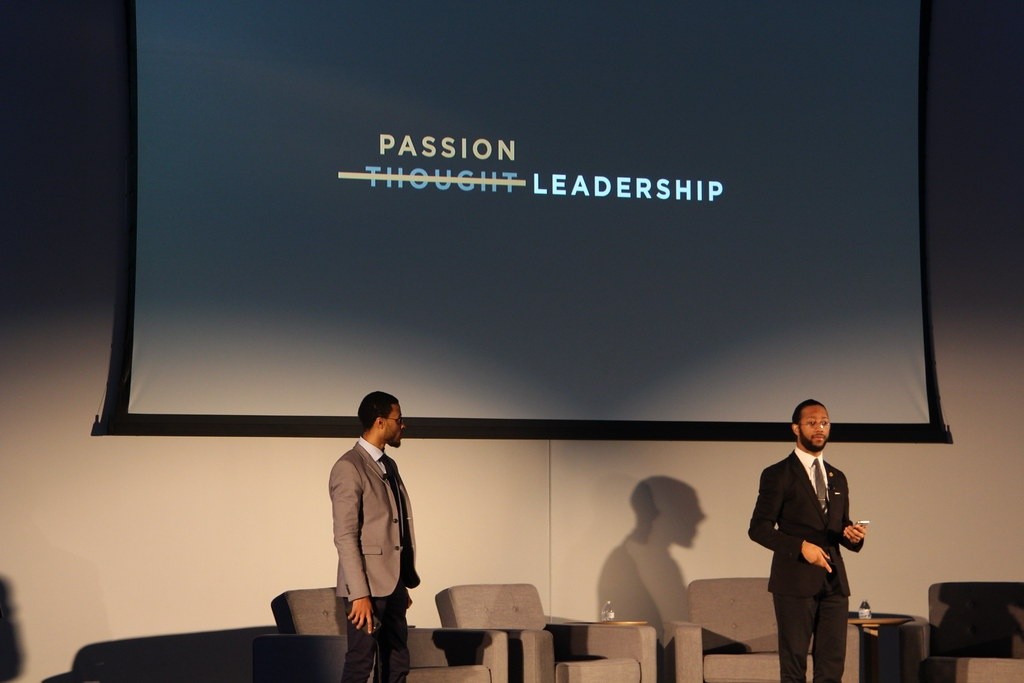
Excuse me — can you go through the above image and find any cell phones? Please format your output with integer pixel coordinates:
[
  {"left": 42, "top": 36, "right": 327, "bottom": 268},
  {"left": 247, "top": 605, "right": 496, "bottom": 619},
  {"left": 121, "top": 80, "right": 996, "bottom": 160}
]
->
[
  {"left": 345, "top": 602, "right": 382, "bottom": 637},
  {"left": 843, "top": 520, "right": 870, "bottom": 537}
]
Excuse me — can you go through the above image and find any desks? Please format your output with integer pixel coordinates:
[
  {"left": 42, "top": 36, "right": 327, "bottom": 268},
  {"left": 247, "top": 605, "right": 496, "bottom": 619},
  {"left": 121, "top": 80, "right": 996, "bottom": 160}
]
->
[{"left": 846, "top": 609, "right": 916, "bottom": 683}]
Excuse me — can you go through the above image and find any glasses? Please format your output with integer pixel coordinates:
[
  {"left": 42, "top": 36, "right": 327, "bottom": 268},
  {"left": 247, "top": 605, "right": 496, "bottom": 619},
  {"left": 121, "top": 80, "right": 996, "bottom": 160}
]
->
[
  {"left": 798, "top": 418, "right": 829, "bottom": 430},
  {"left": 382, "top": 415, "right": 404, "bottom": 425}
]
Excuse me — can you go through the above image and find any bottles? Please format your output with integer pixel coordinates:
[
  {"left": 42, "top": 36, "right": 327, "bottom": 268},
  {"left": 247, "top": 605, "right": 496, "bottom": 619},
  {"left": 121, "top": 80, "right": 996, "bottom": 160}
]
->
[
  {"left": 859, "top": 600, "right": 872, "bottom": 619},
  {"left": 601, "top": 601, "right": 615, "bottom": 622}
]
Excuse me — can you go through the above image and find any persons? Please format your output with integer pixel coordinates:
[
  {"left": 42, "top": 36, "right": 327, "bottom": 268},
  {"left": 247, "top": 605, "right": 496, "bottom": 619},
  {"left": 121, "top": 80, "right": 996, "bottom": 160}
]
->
[
  {"left": 328, "top": 391, "right": 421, "bottom": 683},
  {"left": 747, "top": 399, "right": 867, "bottom": 683}
]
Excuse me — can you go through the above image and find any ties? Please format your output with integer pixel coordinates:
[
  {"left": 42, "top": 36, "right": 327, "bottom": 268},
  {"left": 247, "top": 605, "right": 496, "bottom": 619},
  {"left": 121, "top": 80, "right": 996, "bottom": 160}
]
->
[
  {"left": 812, "top": 458, "right": 828, "bottom": 518},
  {"left": 378, "top": 456, "right": 404, "bottom": 546}
]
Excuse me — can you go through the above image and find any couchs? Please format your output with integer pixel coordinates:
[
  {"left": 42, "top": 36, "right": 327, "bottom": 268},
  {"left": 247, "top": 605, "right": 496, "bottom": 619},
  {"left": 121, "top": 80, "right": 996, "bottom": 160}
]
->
[
  {"left": 671, "top": 577, "right": 860, "bottom": 683},
  {"left": 897, "top": 580, "right": 1024, "bottom": 683},
  {"left": 251, "top": 587, "right": 509, "bottom": 683},
  {"left": 433, "top": 584, "right": 656, "bottom": 683}
]
